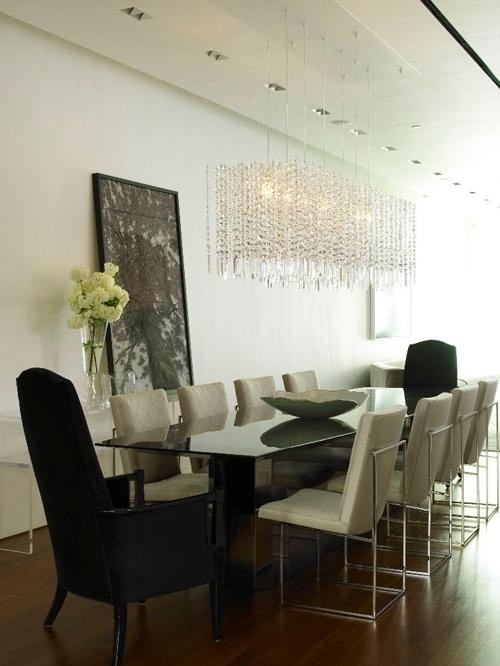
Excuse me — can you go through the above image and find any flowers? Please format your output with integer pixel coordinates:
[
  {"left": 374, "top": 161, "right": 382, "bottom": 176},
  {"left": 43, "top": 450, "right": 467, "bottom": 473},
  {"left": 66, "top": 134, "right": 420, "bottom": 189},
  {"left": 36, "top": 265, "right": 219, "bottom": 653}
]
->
[{"left": 64, "top": 260, "right": 130, "bottom": 395}]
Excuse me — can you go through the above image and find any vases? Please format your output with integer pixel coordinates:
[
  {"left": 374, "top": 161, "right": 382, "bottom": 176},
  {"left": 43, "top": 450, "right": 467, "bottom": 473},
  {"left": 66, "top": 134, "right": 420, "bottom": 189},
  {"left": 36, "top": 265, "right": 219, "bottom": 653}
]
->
[{"left": 78, "top": 322, "right": 109, "bottom": 412}]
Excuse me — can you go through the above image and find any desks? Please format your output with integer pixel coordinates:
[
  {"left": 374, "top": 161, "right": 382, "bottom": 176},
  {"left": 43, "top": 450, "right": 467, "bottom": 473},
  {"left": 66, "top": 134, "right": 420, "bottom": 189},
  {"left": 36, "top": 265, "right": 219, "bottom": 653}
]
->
[{"left": 0, "top": 409, "right": 118, "bottom": 554}]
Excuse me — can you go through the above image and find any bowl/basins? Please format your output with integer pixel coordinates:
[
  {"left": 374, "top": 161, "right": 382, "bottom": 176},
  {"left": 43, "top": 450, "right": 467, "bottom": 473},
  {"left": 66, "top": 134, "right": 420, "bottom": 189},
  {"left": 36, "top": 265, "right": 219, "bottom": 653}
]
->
[{"left": 261, "top": 389, "right": 370, "bottom": 420}]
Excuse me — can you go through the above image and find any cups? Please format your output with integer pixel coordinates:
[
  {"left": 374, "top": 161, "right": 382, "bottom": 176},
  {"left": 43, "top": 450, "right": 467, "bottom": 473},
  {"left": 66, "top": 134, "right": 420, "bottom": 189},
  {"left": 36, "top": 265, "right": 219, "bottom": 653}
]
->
[{"left": 99, "top": 371, "right": 138, "bottom": 409}]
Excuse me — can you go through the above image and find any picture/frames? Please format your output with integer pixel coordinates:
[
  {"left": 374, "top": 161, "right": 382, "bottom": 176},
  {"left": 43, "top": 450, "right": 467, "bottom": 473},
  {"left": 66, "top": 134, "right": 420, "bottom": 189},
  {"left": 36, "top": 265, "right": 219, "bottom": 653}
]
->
[{"left": 90, "top": 172, "right": 195, "bottom": 397}]
